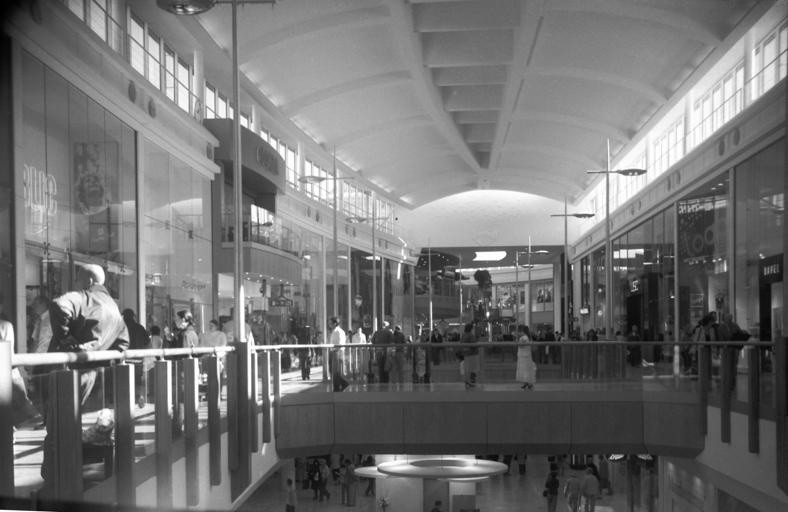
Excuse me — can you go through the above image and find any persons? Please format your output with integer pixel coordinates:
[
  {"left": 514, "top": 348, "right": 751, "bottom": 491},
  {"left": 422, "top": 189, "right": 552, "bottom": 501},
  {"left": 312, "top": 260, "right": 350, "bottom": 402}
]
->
[
  {"left": 432, "top": 500, "right": 442, "bottom": 510},
  {"left": 475, "top": 454, "right": 615, "bottom": 512},
  {"left": 286, "top": 453, "right": 376, "bottom": 510},
  {"left": 0, "top": 263, "right": 325, "bottom": 482},
  {"left": 326, "top": 275, "right": 745, "bottom": 392}
]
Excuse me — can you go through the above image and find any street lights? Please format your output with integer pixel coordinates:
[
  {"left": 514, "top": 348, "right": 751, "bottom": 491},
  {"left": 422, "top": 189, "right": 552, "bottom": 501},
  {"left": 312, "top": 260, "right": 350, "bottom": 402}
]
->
[
  {"left": 410, "top": 245, "right": 439, "bottom": 342},
  {"left": 298, "top": 143, "right": 353, "bottom": 383},
  {"left": 157, "top": 0, "right": 276, "bottom": 341},
  {"left": 445, "top": 256, "right": 469, "bottom": 323},
  {"left": 550, "top": 197, "right": 594, "bottom": 340},
  {"left": 587, "top": 138, "right": 646, "bottom": 340},
  {"left": 516, "top": 235, "right": 549, "bottom": 326},
  {"left": 347, "top": 200, "right": 385, "bottom": 334}
]
